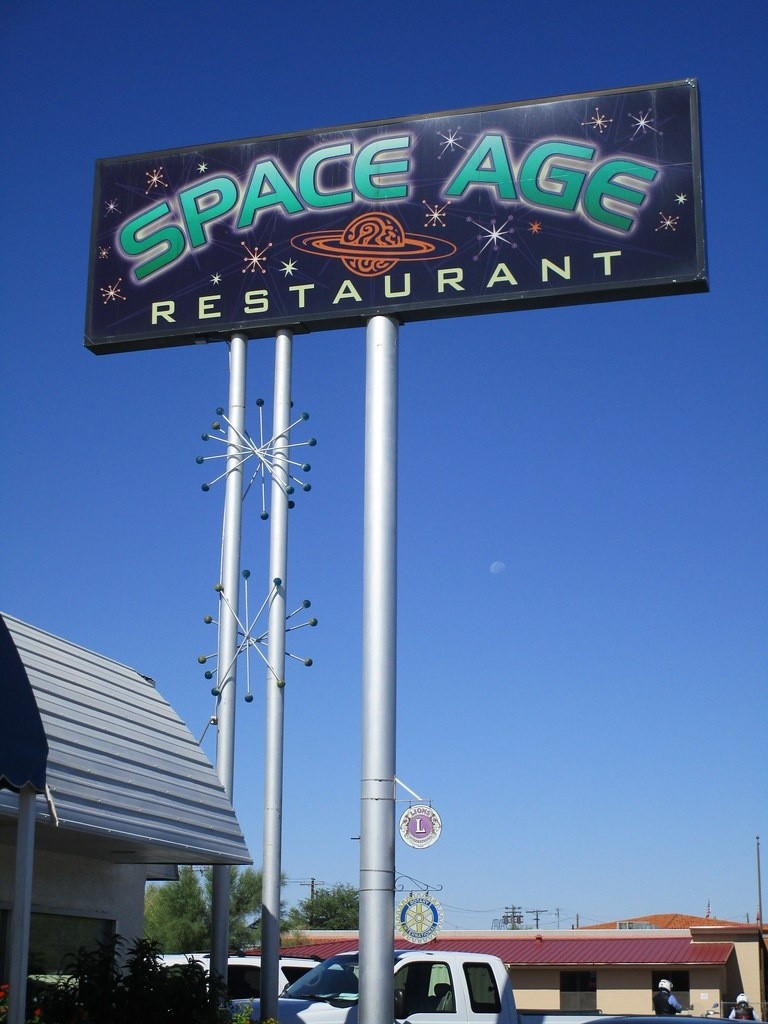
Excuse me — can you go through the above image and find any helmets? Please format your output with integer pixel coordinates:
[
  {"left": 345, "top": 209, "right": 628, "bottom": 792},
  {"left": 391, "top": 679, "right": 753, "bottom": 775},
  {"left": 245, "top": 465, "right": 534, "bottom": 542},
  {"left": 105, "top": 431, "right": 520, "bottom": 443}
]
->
[
  {"left": 658, "top": 979, "right": 673, "bottom": 992},
  {"left": 736, "top": 994, "right": 748, "bottom": 1006}
]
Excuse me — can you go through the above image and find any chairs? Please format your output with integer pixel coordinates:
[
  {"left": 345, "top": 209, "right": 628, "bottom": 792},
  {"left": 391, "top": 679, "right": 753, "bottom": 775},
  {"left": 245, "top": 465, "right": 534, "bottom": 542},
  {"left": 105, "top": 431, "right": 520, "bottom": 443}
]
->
[{"left": 395, "top": 982, "right": 451, "bottom": 1021}]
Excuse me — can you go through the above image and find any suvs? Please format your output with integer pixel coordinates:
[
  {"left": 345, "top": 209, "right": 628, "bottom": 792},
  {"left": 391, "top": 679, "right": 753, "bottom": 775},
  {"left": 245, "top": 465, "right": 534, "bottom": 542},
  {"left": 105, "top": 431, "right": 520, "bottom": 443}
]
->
[{"left": 172, "top": 952, "right": 327, "bottom": 1024}]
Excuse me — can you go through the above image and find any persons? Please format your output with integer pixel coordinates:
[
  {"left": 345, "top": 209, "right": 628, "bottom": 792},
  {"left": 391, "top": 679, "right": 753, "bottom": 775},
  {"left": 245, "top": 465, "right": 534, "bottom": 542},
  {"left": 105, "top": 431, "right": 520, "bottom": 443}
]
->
[
  {"left": 653, "top": 979, "right": 683, "bottom": 1016},
  {"left": 728, "top": 994, "right": 761, "bottom": 1021}
]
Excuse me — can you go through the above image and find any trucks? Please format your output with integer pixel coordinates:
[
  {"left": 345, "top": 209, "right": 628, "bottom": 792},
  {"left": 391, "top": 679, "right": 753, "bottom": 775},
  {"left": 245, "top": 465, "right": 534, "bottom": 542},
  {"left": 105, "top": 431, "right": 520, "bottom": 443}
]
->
[{"left": 277, "top": 949, "right": 688, "bottom": 1024}]
